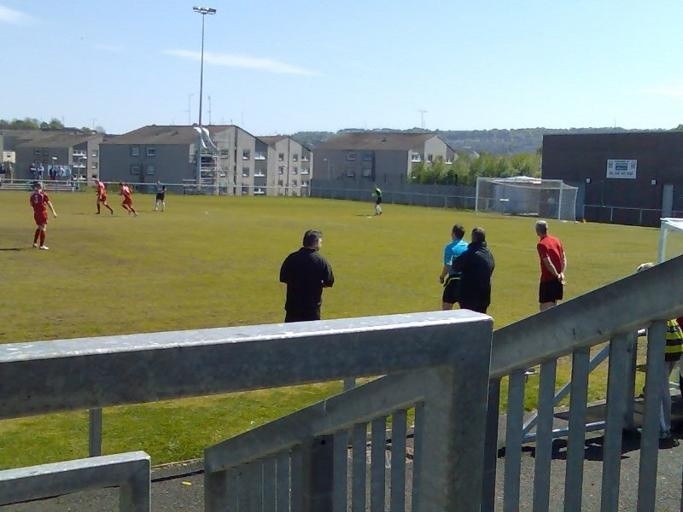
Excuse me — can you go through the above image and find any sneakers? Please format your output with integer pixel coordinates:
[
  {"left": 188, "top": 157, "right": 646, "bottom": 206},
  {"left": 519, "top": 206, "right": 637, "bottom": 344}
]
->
[
  {"left": 128, "top": 209, "right": 139, "bottom": 216},
  {"left": 33, "top": 243, "right": 50, "bottom": 250}
]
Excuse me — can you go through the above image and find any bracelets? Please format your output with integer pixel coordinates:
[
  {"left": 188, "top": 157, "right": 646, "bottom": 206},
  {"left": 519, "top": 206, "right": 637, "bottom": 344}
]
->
[
  {"left": 440, "top": 277, "right": 444, "bottom": 280},
  {"left": 554, "top": 274, "right": 559, "bottom": 279}
]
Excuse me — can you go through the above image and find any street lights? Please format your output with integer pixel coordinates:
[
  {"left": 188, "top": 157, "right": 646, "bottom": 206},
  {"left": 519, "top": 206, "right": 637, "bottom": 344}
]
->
[{"left": 193, "top": 6, "right": 216, "bottom": 193}]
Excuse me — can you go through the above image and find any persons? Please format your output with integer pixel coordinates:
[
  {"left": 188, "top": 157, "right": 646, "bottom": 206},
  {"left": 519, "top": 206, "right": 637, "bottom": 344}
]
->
[
  {"left": 372, "top": 185, "right": 384, "bottom": 216},
  {"left": 439, "top": 223, "right": 470, "bottom": 310},
  {"left": 635, "top": 262, "right": 682, "bottom": 447},
  {"left": 674, "top": 317, "right": 683, "bottom": 399},
  {"left": 0, "top": 159, "right": 71, "bottom": 184},
  {"left": 535, "top": 220, "right": 567, "bottom": 313},
  {"left": 279, "top": 229, "right": 335, "bottom": 323},
  {"left": 151, "top": 180, "right": 168, "bottom": 211},
  {"left": 30, "top": 184, "right": 58, "bottom": 250},
  {"left": 119, "top": 182, "right": 138, "bottom": 217},
  {"left": 94, "top": 180, "right": 114, "bottom": 215},
  {"left": 454, "top": 226, "right": 495, "bottom": 314}
]
